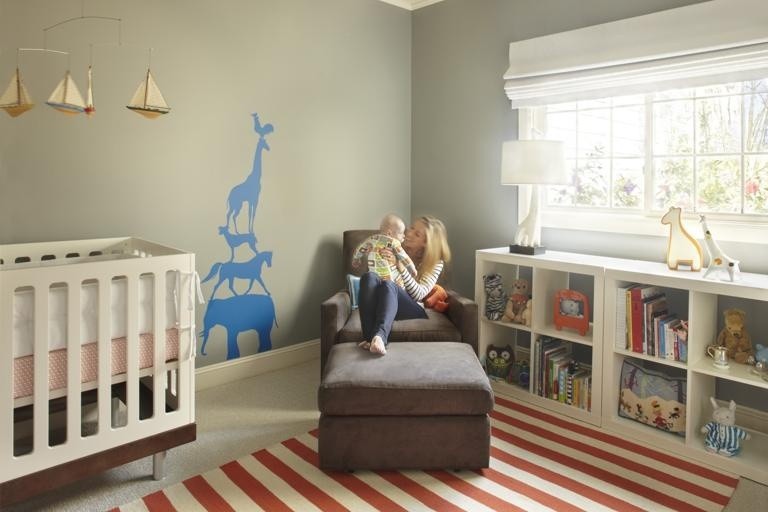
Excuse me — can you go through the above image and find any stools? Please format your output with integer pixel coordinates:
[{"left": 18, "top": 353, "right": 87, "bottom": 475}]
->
[{"left": 316, "top": 339, "right": 494, "bottom": 471}]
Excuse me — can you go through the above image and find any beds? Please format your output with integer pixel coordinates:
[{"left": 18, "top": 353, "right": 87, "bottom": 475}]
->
[{"left": 1, "top": 234, "right": 197, "bottom": 509}]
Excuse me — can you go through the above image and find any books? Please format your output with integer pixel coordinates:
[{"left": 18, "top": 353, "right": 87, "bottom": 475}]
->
[
  {"left": 616, "top": 283, "right": 688, "bottom": 364},
  {"left": 534, "top": 334, "right": 592, "bottom": 412}
]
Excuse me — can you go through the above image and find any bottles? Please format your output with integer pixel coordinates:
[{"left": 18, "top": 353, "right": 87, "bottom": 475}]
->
[
  {"left": 519, "top": 359, "right": 529, "bottom": 388},
  {"left": 756, "top": 360, "right": 768, "bottom": 372}
]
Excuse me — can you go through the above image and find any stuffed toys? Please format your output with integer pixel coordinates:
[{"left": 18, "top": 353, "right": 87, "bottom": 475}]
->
[
  {"left": 747, "top": 343, "right": 768, "bottom": 381},
  {"left": 519, "top": 299, "right": 532, "bottom": 330},
  {"left": 501, "top": 278, "right": 530, "bottom": 324},
  {"left": 507, "top": 358, "right": 530, "bottom": 389},
  {"left": 706, "top": 307, "right": 756, "bottom": 365},
  {"left": 483, "top": 342, "right": 516, "bottom": 381},
  {"left": 699, "top": 395, "right": 753, "bottom": 457},
  {"left": 483, "top": 272, "right": 509, "bottom": 323}
]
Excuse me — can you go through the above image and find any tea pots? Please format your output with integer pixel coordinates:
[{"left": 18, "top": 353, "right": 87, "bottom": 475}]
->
[{"left": 708, "top": 345, "right": 730, "bottom": 365}]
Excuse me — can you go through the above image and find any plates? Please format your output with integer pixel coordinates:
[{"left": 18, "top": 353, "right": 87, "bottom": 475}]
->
[{"left": 713, "top": 362, "right": 731, "bottom": 369}]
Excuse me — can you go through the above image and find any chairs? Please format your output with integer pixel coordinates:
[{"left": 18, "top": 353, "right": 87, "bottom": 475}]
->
[{"left": 319, "top": 230, "right": 479, "bottom": 387}]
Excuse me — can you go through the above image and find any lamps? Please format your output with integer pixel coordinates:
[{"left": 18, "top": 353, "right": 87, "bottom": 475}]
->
[{"left": 498, "top": 137, "right": 571, "bottom": 255}]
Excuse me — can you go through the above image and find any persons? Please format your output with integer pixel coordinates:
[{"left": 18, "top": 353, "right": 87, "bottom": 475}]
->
[
  {"left": 560, "top": 299, "right": 584, "bottom": 319},
  {"left": 352, "top": 213, "right": 418, "bottom": 292},
  {"left": 356, "top": 215, "right": 452, "bottom": 355}
]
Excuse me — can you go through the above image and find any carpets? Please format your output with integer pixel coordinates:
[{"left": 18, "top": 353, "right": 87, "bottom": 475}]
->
[{"left": 97, "top": 394, "right": 740, "bottom": 511}]
